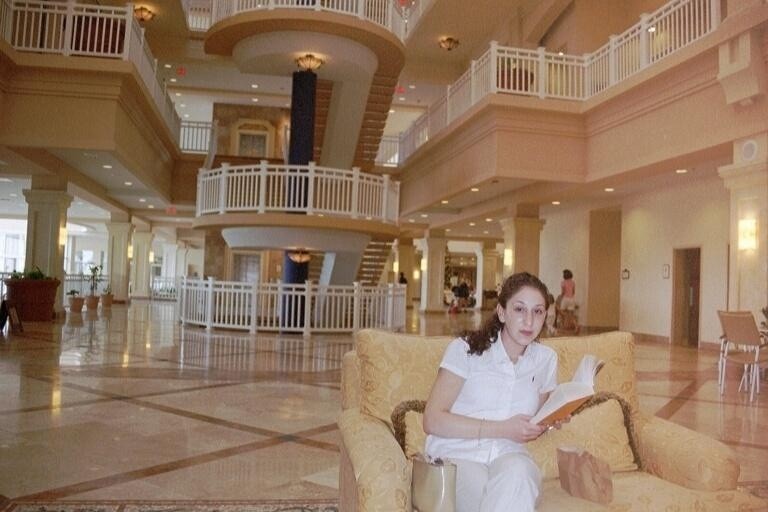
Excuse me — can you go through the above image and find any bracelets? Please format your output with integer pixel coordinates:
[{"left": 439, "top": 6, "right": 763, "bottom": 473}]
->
[{"left": 478, "top": 417, "right": 486, "bottom": 439}]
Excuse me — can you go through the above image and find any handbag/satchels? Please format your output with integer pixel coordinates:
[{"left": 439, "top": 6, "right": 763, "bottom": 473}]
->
[
  {"left": 411, "top": 453, "right": 457, "bottom": 511},
  {"left": 556, "top": 448, "right": 611, "bottom": 505}
]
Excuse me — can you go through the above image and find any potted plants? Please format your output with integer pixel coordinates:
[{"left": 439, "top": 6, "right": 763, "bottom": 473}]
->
[{"left": 2, "top": 264, "right": 114, "bottom": 322}]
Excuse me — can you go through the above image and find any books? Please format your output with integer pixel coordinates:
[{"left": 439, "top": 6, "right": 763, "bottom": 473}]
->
[{"left": 529, "top": 354, "right": 606, "bottom": 430}]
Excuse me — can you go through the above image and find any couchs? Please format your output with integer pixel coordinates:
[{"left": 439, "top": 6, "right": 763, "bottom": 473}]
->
[{"left": 340, "top": 329, "right": 766, "bottom": 510}]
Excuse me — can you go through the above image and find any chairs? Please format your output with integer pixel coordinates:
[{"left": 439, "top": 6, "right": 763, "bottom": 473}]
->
[{"left": 717, "top": 310, "right": 766, "bottom": 402}]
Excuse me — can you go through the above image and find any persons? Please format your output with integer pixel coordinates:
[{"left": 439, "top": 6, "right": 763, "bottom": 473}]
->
[
  {"left": 449, "top": 272, "right": 470, "bottom": 314},
  {"left": 399, "top": 272, "right": 408, "bottom": 284},
  {"left": 421, "top": 271, "right": 571, "bottom": 512},
  {"left": 496, "top": 283, "right": 502, "bottom": 297},
  {"left": 545, "top": 294, "right": 565, "bottom": 337},
  {"left": 559, "top": 269, "right": 580, "bottom": 335}
]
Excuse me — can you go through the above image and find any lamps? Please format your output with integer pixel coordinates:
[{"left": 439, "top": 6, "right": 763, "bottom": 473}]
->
[
  {"left": 134, "top": 7, "right": 154, "bottom": 22},
  {"left": 288, "top": 251, "right": 312, "bottom": 263},
  {"left": 294, "top": 53, "right": 326, "bottom": 71},
  {"left": 438, "top": 35, "right": 460, "bottom": 52}
]
